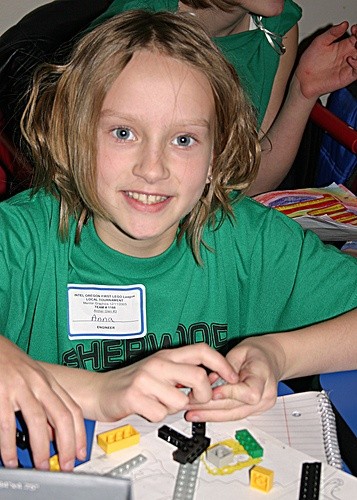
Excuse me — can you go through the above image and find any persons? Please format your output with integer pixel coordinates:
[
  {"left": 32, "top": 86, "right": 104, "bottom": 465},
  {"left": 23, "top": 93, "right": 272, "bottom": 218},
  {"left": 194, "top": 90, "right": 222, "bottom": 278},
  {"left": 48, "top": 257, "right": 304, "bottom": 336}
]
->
[
  {"left": 1, "top": 1, "right": 356, "bottom": 198},
  {"left": 0, "top": 334, "right": 89, "bottom": 476},
  {"left": 0, "top": 11, "right": 357, "bottom": 422}
]
